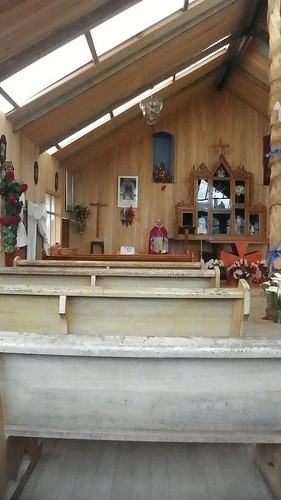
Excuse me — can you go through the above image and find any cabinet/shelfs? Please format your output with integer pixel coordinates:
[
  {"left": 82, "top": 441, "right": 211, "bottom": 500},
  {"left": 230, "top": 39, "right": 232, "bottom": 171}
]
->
[{"left": 174, "top": 151, "right": 267, "bottom": 245}]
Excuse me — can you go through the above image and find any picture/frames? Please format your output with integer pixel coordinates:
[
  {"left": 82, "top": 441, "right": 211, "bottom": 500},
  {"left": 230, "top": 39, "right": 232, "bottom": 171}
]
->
[{"left": 117, "top": 175, "right": 139, "bottom": 208}]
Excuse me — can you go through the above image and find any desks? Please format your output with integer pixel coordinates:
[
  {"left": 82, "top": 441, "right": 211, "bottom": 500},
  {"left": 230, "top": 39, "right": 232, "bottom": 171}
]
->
[{"left": 90, "top": 241, "right": 105, "bottom": 254}]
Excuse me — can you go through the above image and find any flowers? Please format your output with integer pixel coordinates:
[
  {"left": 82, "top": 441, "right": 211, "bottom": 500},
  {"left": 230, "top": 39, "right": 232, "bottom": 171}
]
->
[
  {"left": 225, "top": 257, "right": 266, "bottom": 284},
  {"left": 0, "top": 169, "right": 28, "bottom": 253},
  {"left": 205, "top": 259, "right": 226, "bottom": 280},
  {"left": 260, "top": 271, "right": 281, "bottom": 323}
]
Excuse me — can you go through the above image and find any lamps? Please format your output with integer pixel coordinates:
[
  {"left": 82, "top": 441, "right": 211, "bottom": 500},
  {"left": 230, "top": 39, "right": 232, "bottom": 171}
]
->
[{"left": 139, "top": 84, "right": 164, "bottom": 126}]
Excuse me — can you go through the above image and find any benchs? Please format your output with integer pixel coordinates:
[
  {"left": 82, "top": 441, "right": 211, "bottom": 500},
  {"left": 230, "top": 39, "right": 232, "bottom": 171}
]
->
[
  {"left": 0, "top": 250, "right": 220, "bottom": 288},
  {"left": 0, "top": 330, "right": 281, "bottom": 500},
  {"left": 0, "top": 279, "right": 251, "bottom": 336}
]
